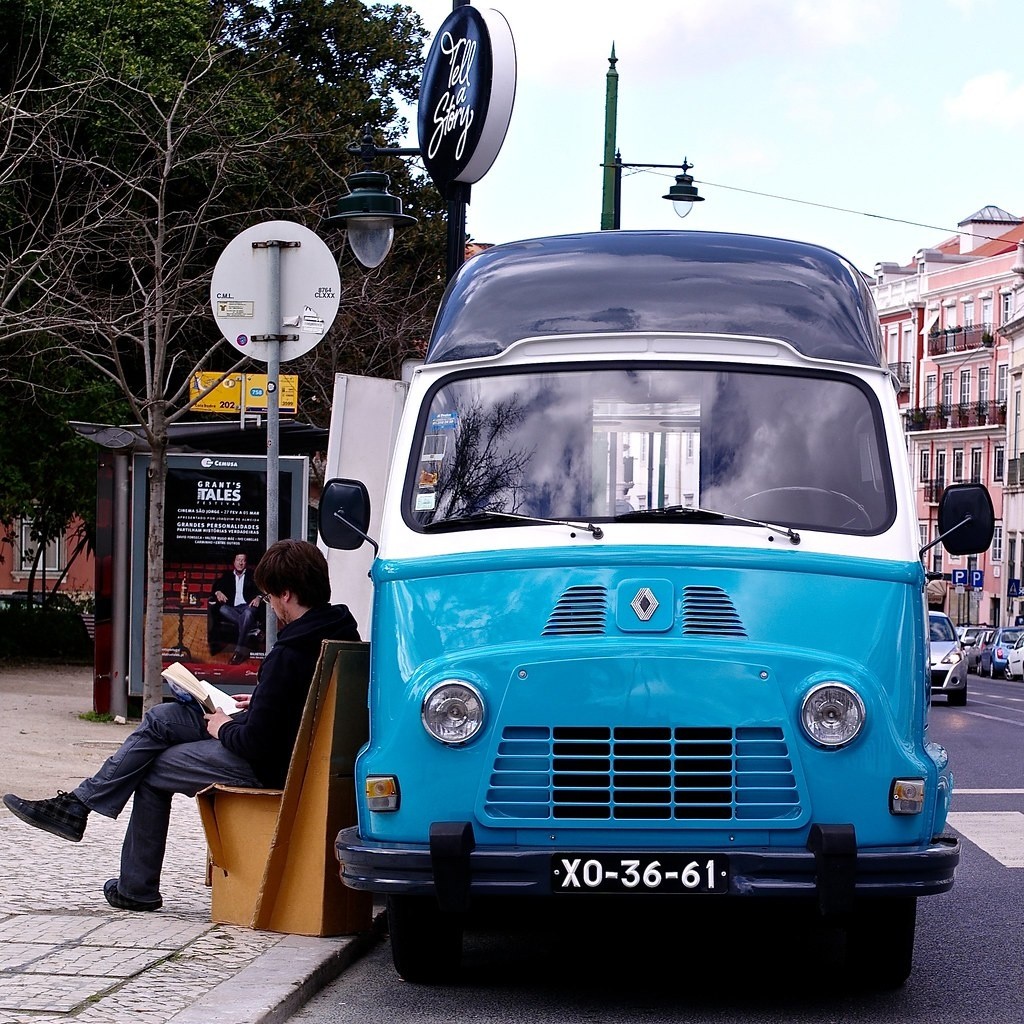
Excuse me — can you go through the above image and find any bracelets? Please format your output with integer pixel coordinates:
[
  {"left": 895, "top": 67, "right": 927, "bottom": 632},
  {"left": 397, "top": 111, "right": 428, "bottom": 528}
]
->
[{"left": 257, "top": 595, "right": 263, "bottom": 599}]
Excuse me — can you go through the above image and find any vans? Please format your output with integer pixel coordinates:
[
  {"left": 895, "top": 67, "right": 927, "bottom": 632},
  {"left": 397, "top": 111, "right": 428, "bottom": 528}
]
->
[{"left": 316, "top": 230, "right": 998, "bottom": 1001}]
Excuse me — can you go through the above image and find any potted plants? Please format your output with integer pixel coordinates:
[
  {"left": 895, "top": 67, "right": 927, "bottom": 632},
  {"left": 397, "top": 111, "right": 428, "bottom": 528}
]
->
[
  {"left": 938, "top": 409, "right": 948, "bottom": 429},
  {"left": 902, "top": 409, "right": 925, "bottom": 430},
  {"left": 982, "top": 332, "right": 994, "bottom": 347},
  {"left": 975, "top": 407, "right": 987, "bottom": 425},
  {"left": 997, "top": 404, "right": 1006, "bottom": 424}
]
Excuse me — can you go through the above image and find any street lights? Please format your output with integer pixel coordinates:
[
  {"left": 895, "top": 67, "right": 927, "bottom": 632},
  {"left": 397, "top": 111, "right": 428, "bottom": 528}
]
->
[{"left": 597, "top": 153, "right": 704, "bottom": 233}]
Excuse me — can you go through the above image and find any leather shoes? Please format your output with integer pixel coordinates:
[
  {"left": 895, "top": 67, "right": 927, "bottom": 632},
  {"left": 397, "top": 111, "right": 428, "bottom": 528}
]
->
[
  {"left": 229, "top": 652, "right": 250, "bottom": 666},
  {"left": 254, "top": 629, "right": 266, "bottom": 641}
]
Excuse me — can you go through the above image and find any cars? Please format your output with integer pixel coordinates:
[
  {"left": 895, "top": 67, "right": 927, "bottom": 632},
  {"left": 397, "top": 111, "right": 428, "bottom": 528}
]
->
[
  {"left": 955, "top": 624, "right": 1024, "bottom": 682},
  {"left": 929, "top": 609, "right": 977, "bottom": 707}
]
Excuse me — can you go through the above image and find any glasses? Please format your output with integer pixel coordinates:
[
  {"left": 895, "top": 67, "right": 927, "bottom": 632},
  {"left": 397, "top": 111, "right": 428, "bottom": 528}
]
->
[{"left": 259, "top": 591, "right": 272, "bottom": 604}]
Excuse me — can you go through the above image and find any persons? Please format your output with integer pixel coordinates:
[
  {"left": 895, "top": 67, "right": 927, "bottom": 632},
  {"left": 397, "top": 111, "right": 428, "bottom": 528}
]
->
[
  {"left": 212, "top": 554, "right": 265, "bottom": 665},
  {"left": 3, "top": 539, "right": 362, "bottom": 911}
]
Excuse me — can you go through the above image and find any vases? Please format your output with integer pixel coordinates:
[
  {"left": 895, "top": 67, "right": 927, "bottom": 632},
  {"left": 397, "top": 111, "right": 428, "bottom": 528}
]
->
[
  {"left": 922, "top": 419, "right": 931, "bottom": 430},
  {"left": 958, "top": 415, "right": 969, "bottom": 427}
]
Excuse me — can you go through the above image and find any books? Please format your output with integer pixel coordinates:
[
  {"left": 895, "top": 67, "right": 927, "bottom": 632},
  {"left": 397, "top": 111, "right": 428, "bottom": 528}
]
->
[{"left": 161, "top": 662, "right": 248, "bottom": 719}]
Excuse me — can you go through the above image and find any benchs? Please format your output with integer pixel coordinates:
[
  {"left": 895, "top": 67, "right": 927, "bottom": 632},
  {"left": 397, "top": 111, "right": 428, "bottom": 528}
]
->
[{"left": 192, "top": 638, "right": 371, "bottom": 939}]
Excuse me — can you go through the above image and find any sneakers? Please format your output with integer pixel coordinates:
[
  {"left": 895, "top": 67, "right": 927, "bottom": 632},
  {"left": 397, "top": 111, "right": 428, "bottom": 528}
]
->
[
  {"left": 104, "top": 878, "right": 163, "bottom": 911},
  {"left": 3, "top": 790, "right": 88, "bottom": 842}
]
